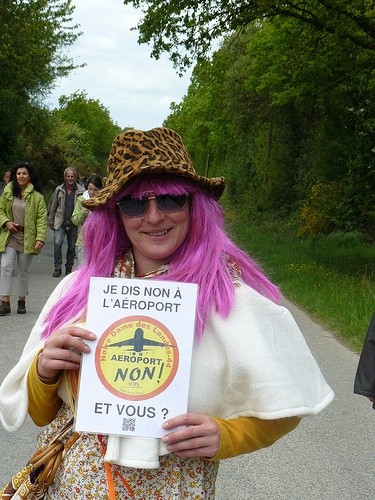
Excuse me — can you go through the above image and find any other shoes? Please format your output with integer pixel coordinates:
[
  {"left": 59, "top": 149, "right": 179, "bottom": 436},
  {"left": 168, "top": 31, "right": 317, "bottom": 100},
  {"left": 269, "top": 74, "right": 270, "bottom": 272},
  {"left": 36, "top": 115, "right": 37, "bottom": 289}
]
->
[
  {"left": 52, "top": 269, "right": 62, "bottom": 277},
  {"left": 17, "top": 300, "right": 26, "bottom": 314},
  {"left": 0, "top": 300, "right": 11, "bottom": 316},
  {"left": 66, "top": 267, "right": 72, "bottom": 276}
]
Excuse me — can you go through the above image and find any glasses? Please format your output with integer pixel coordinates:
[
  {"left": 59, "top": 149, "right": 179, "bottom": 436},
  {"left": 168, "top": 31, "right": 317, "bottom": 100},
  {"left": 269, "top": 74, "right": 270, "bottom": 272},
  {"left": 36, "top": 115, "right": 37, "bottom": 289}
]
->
[{"left": 112, "top": 195, "right": 196, "bottom": 217}]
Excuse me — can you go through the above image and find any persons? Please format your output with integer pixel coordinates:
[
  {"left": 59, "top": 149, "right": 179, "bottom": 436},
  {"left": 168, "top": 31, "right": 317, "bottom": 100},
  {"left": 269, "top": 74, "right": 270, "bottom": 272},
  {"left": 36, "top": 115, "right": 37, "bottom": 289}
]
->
[{"left": 0, "top": 127, "right": 335, "bottom": 500}]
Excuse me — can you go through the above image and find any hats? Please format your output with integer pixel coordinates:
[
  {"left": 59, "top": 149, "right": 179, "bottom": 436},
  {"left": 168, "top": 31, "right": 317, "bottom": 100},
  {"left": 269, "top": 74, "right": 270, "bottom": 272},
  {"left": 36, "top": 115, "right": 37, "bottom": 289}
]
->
[{"left": 82, "top": 129, "right": 227, "bottom": 213}]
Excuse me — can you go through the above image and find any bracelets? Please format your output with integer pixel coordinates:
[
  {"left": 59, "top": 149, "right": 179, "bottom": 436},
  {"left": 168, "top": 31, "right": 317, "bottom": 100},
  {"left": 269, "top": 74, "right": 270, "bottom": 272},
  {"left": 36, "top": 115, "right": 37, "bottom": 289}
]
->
[{"left": 36, "top": 352, "right": 60, "bottom": 384}]
